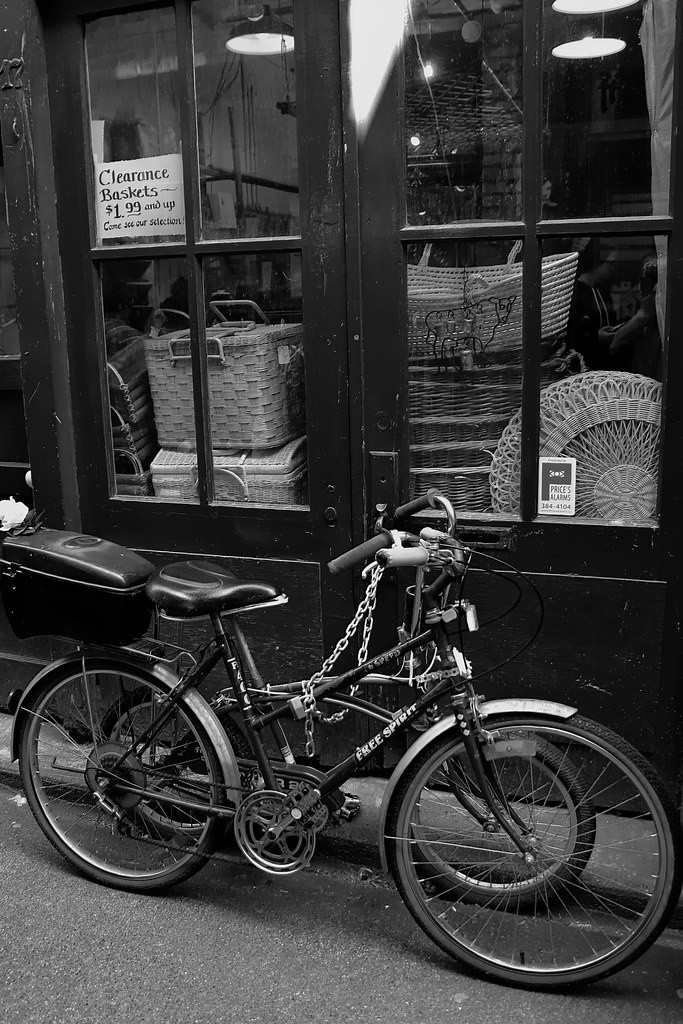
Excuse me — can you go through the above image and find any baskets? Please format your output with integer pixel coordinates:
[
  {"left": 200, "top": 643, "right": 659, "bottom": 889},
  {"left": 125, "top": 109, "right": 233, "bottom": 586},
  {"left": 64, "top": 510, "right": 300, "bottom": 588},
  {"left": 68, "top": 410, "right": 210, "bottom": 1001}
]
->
[{"left": 406, "top": 238, "right": 579, "bottom": 511}]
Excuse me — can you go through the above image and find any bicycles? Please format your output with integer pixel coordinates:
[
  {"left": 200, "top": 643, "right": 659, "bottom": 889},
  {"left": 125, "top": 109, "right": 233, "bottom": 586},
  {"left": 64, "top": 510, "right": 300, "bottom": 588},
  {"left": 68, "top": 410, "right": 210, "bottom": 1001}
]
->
[{"left": 8, "top": 487, "right": 683, "bottom": 992}]
